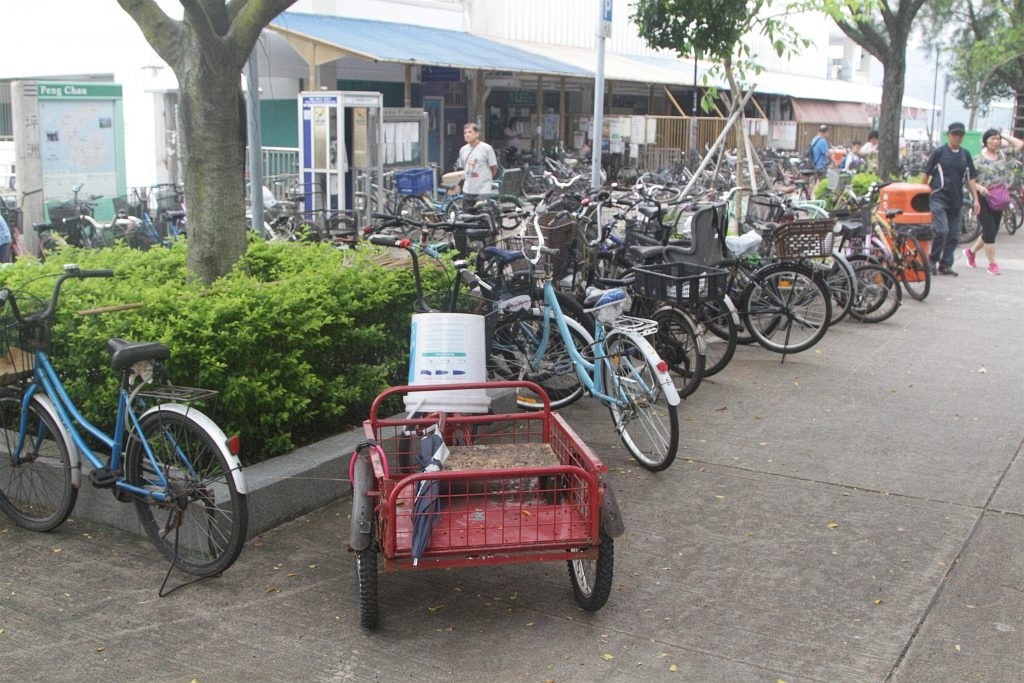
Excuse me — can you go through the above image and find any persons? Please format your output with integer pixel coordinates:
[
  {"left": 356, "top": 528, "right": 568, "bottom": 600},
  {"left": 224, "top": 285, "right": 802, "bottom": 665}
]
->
[
  {"left": 844, "top": 140, "right": 867, "bottom": 175},
  {"left": 963, "top": 127, "right": 1023, "bottom": 276},
  {"left": 859, "top": 129, "right": 880, "bottom": 173},
  {"left": 451, "top": 123, "right": 498, "bottom": 263},
  {"left": 921, "top": 122, "right": 981, "bottom": 276},
  {"left": 810, "top": 124, "right": 832, "bottom": 181}
]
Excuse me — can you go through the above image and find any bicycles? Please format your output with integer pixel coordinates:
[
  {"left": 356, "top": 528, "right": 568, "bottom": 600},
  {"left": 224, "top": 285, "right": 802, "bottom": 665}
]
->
[
  {"left": 0, "top": 263, "right": 251, "bottom": 597},
  {"left": 0, "top": 188, "right": 47, "bottom": 267},
  {"left": 30, "top": 138, "right": 1024, "bottom": 403},
  {"left": 484, "top": 198, "right": 681, "bottom": 471}
]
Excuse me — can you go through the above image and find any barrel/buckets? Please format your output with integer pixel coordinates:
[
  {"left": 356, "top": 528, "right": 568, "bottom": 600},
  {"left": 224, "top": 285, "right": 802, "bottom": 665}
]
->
[{"left": 402, "top": 313, "right": 491, "bottom": 437}]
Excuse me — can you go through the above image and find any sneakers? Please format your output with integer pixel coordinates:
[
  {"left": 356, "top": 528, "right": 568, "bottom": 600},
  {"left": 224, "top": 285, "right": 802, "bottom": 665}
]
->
[
  {"left": 963, "top": 248, "right": 976, "bottom": 267},
  {"left": 986, "top": 262, "right": 1000, "bottom": 275},
  {"left": 938, "top": 262, "right": 958, "bottom": 276},
  {"left": 928, "top": 259, "right": 937, "bottom": 275}
]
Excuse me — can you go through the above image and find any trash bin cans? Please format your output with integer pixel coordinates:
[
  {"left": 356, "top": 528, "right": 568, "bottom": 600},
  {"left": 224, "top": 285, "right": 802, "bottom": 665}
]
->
[{"left": 876, "top": 182, "right": 933, "bottom": 282}]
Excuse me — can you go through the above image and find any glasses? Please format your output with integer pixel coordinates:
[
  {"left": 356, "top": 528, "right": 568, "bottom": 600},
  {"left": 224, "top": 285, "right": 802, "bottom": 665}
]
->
[{"left": 950, "top": 132, "right": 963, "bottom": 137}]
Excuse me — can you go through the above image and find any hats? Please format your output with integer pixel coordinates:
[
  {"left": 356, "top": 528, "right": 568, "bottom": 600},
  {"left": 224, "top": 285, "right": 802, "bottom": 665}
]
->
[{"left": 949, "top": 122, "right": 964, "bottom": 134}]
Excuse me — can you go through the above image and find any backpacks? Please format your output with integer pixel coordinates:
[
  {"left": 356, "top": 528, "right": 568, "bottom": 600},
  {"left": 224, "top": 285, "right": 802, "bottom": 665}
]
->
[{"left": 799, "top": 136, "right": 828, "bottom": 175}]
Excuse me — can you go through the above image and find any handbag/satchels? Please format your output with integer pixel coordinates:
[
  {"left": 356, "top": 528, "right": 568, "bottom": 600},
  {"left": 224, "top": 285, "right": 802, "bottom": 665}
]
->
[{"left": 984, "top": 184, "right": 1011, "bottom": 211}]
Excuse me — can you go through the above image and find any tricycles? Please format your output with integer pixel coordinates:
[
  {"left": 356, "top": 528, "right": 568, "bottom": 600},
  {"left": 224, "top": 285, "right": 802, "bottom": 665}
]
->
[{"left": 348, "top": 234, "right": 625, "bottom": 631}]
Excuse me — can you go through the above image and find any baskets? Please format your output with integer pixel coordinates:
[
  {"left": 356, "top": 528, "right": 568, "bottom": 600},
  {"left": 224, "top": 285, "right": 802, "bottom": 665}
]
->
[
  {"left": 414, "top": 290, "right": 499, "bottom": 365},
  {"left": 0, "top": 207, "right": 24, "bottom": 235},
  {"left": 48, "top": 199, "right": 93, "bottom": 236},
  {"left": 506, "top": 200, "right": 582, "bottom": 272},
  {"left": 479, "top": 274, "right": 532, "bottom": 322},
  {"left": 631, "top": 261, "right": 730, "bottom": 306},
  {"left": 624, "top": 220, "right": 663, "bottom": 258},
  {"left": 745, "top": 194, "right": 784, "bottom": 225},
  {"left": 112, "top": 195, "right": 144, "bottom": 219},
  {"left": 775, "top": 220, "right": 835, "bottom": 259},
  {"left": 0, "top": 314, "right": 51, "bottom": 386},
  {"left": 395, "top": 168, "right": 434, "bottom": 195},
  {"left": 155, "top": 190, "right": 178, "bottom": 213}
]
